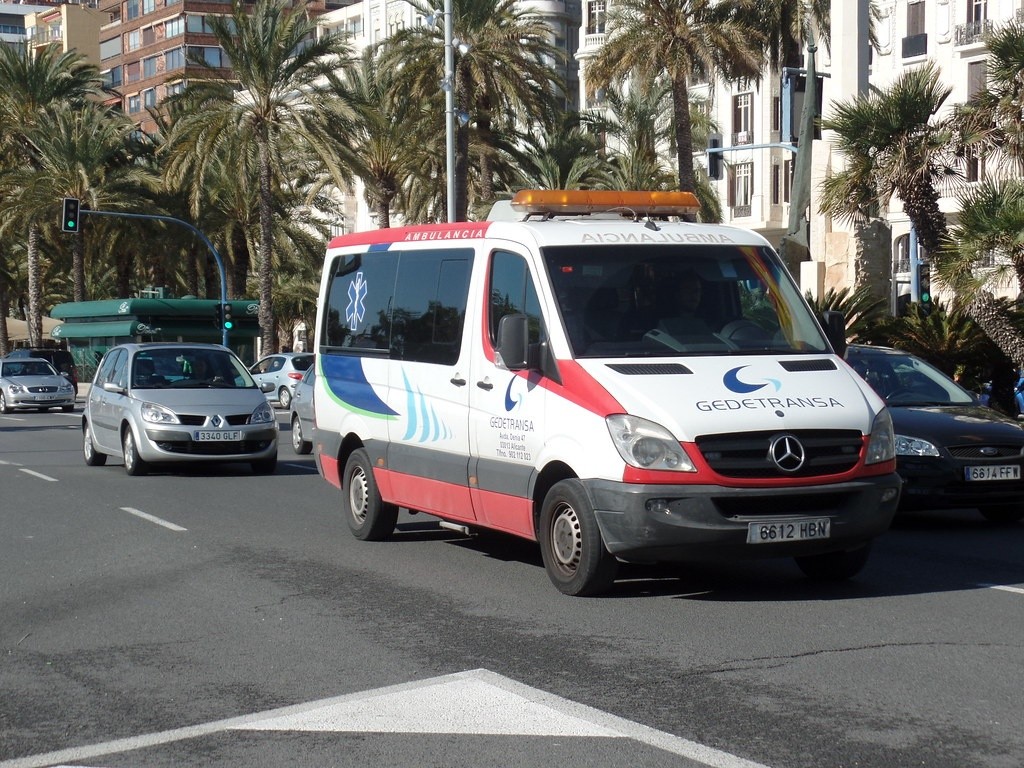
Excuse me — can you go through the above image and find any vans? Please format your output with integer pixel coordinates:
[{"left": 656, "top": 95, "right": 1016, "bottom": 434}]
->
[
  {"left": 1, "top": 346, "right": 78, "bottom": 411},
  {"left": 313, "top": 190, "right": 904, "bottom": 596}
]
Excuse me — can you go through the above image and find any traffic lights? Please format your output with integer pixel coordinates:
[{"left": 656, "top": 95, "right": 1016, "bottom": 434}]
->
[
  {"left": 223, "top": 303, "right": 233, "bottom": 331},
  {"left": 212, "top": 301, "right": 223, "bottom": 332},
  {"left": 63, "top": 198, "right": 80, "bottom": 232},
  {"left": 916, "top": 263, "right": 931, "bottom": 304}
]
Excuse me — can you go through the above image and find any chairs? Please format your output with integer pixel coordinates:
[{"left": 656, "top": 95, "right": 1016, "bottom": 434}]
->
[
  {"left": 189, "top": 361, "right": 218, "bottom": 384},
  {"left": 3, "top": 367, "right": 11, "bottom": 376},
  {"left": 135, "top": 359, "right": 162, "bottom": 387},
  {"left": 866, "top": 361, "right": 904, "bottom": 400}
]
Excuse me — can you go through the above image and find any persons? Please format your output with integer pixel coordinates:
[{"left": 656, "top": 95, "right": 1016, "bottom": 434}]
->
[
  {"left": 674, "top": 267, "right": 729, "bottom": 333},
  {"left": 189, "top": 357, "right": 217, "bottom": 380}
]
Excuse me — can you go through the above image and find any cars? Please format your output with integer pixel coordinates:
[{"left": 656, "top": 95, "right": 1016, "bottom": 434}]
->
[
  {"left": 0, "top": 357, "right": 76, "bottom": 414},
  {"left": 81, "top": 342, "right": 279, "bottom": 477},
  {"left": 234, "top": 352, "right": 314, "bottom": 410},
  {"left": 842, "top": 344, "right": 1024, "bottom": 530},
  {"left": 289, "top": 363, "right": 316, "bottom": 455}
]
